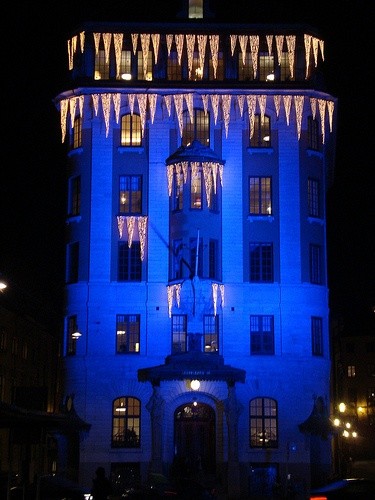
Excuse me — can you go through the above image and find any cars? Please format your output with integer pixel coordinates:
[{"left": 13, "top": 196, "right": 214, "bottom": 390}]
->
[{"left": 306, "top": 478, "right": 375, "bottom": 500}]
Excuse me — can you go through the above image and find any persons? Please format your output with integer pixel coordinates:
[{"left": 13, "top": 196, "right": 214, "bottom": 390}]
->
[{"left": 89, "top": 465, "right": 109, "bottom": 500}]
